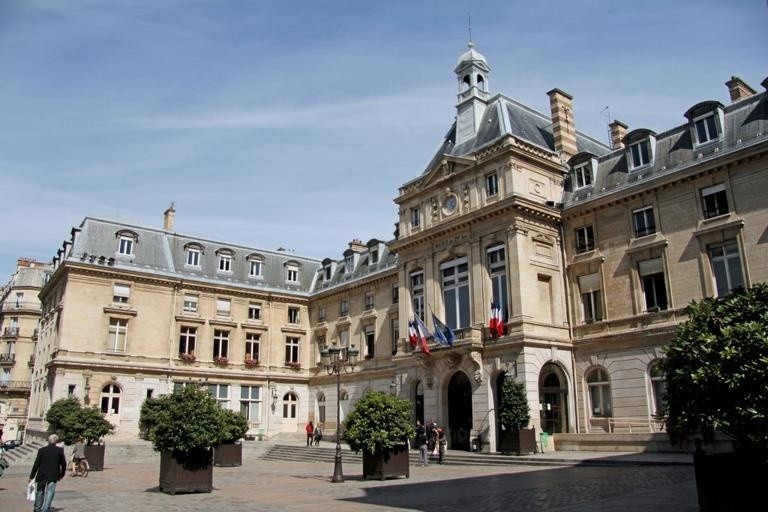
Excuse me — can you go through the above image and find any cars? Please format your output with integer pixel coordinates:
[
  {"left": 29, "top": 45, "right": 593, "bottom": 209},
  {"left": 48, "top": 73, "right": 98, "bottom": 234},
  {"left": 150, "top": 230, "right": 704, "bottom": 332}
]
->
[{"left": 1, "top": 440, "right": 23, "bottom": 451}]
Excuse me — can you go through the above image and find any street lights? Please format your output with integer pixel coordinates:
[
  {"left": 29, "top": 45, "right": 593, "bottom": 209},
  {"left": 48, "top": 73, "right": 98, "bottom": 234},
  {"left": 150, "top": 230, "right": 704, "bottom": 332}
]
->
[{"left": 321, "top": 344, "right": 358, "bottom": 482}]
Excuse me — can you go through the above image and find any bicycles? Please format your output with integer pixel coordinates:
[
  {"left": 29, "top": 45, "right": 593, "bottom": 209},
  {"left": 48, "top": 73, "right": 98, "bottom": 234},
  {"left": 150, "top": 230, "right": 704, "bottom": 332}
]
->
[{"left": 68, "top": 452, "right": 89, "bottom": 478}]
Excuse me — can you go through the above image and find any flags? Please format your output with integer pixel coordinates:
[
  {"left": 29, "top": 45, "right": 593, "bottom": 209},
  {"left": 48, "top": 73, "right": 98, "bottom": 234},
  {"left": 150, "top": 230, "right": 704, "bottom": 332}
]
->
[
  {"left": 414, "top": 314, "right": 433, "bottom": 354},
  {"left": 431, "top": 311, "right": 457, "bottom": 347},
  {"left": 494, "top": 301, "right": 505, "bottom": 340},
  {"left": 407, "top": 318, "right": 419, "bottom": 350},
  {"left": 488, "top": 300, "right": 496, "bottom": 338}
]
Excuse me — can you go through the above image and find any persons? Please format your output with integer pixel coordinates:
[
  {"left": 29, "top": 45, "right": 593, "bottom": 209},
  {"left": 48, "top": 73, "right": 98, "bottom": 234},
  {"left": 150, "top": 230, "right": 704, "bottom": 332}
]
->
[
  {"left": 305, "top": 420, "right": 314, "bottom": 445},
  {"left": 70, "top": 435, "right": 86, "bottom": 477},
  {"left": 313, "top": 422, "right": 322, "bottom": 446},
  {"left": 26, "top": 432, "right": 67, "bottom": 511},
  {"left": 412, "top": 415, "right": 449, "bottom": 467}
]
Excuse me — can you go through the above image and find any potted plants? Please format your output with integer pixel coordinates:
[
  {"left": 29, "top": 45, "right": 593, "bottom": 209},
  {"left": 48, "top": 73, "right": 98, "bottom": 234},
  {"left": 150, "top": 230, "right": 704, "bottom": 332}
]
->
[
  {"left": 136, "top": 380, "right": 250, "bottom": 497},
  {"left": 337, "top": 386, "right": 420, "bottom": 481},
  {"left": 44, "top": 396, "right": 115, "bottom": 471},
  {"left": 494, "top": 376, "right": 538, "bottom": 458},
  {"left": 646, "top": 277, "right": 768, "bottom": 511}
]
